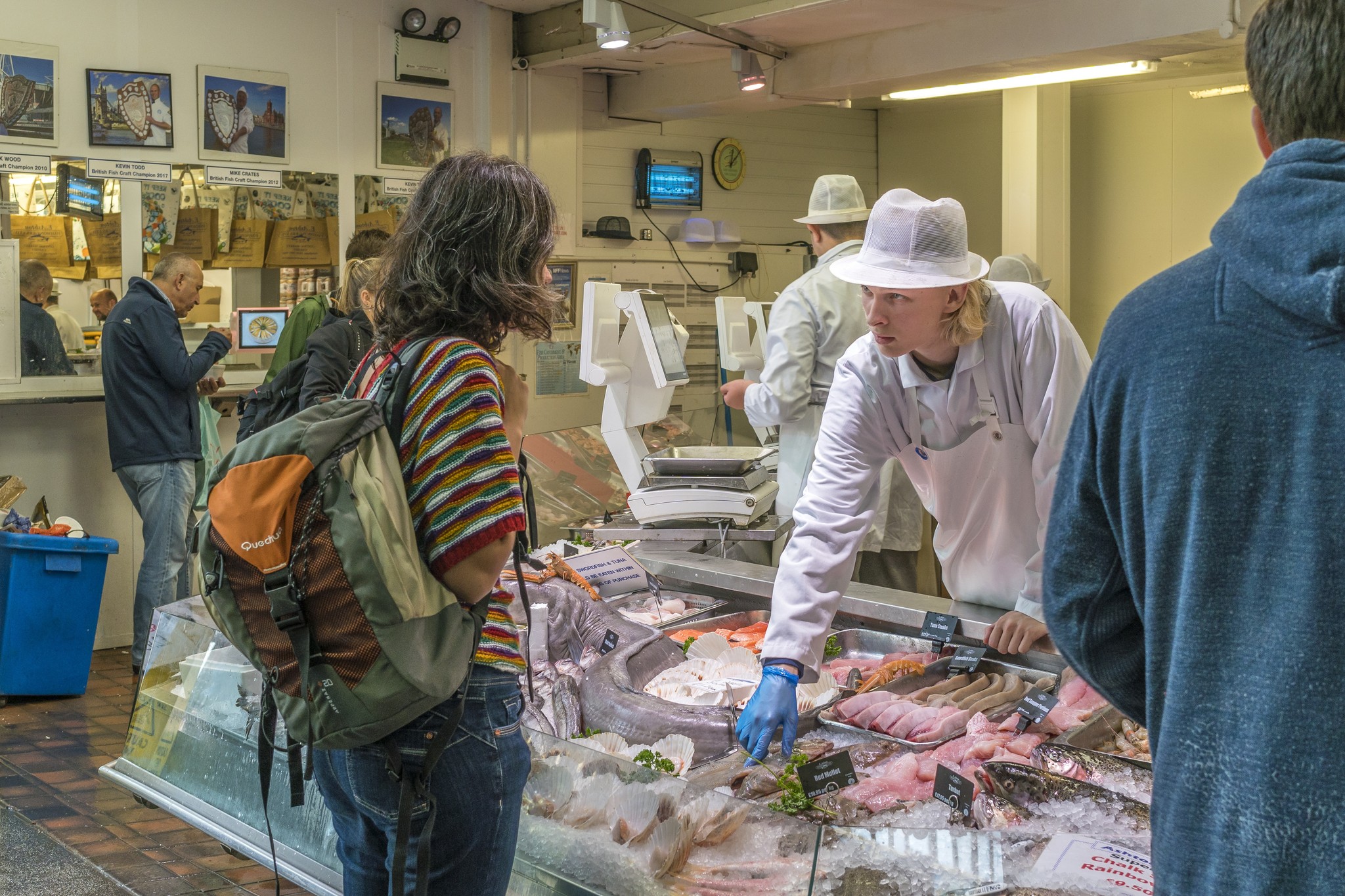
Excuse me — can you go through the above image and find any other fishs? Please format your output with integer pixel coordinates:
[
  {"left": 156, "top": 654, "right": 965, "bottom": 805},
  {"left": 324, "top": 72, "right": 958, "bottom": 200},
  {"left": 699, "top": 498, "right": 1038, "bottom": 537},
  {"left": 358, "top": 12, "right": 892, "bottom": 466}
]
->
[{"left": 970, "top": 742, "right": 1151, "bottom": 836}]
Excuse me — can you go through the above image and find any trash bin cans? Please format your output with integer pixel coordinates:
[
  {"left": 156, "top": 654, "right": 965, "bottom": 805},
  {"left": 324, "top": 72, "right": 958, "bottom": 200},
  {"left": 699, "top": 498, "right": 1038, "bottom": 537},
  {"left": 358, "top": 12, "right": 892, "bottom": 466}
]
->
[{"left": 0, "top": 529, "right": 119, "bottom": 707}]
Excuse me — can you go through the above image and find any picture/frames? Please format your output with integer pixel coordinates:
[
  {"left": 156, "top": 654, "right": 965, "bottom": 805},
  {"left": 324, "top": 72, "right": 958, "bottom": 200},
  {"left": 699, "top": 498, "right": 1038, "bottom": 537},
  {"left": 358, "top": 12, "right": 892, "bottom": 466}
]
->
[
  {"left": 0, "top": 39, "right": 59, "bottom": 147},
  {"left": 376, "top": 80, "right": 455, "bottom": 173},
  {"left": 85, "top": 68, "right": 174, "bottom": 148},
  {"left": 196, "top": 65, "right": 291, "bottom": 165},
  {"left": 541, "top": 260, "right": 578, "bottom": 330},
  {"left": 533, "top": 337, "right": 590, "bottom": 399}
]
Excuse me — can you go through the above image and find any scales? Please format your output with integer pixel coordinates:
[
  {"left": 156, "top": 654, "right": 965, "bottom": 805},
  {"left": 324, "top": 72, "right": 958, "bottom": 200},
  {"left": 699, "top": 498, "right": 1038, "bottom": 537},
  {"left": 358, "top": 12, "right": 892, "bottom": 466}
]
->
[
  {"left": 579, "top": 280, "right": 780, "bottom": 532},
  {"left": 716, "top": 292, "right": 781, "bottom": 473}
]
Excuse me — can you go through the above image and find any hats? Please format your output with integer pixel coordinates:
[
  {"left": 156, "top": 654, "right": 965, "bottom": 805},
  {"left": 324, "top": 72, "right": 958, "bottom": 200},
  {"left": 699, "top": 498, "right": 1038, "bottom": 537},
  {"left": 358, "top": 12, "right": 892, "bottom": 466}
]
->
[
  {"left": 50, "top": 277, "right": 63, "bottom": 296},
  {"left": 828, "top": 188, "right": 990, "bottom": 289},
  {"left": 792, "top": 173, "right": 873, "bottom": 225},
  {"left": 980, "top": 252, "right": 1052, "bottom": 291},
  {"left": 236, "top": 85, "right": 249, "bottom": 98},
  {"left": 588, "top": 216, "right": 742, "bottom": 243}
]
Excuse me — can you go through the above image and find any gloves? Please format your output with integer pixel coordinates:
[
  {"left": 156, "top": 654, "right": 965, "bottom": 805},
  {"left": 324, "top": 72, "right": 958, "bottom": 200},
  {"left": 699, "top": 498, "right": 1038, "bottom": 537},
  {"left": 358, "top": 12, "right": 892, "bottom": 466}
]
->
[{"left": 734, "top": 664, "right": 800, "bottom": 769}]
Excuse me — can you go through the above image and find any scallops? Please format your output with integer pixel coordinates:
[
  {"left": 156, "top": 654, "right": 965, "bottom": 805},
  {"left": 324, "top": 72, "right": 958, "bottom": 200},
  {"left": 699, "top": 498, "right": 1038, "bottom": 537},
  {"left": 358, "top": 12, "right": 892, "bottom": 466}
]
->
[
  {"left": 645, "top": 631, "right": 843, "bottom": 716},
  {"left": 522, "top": 731, "right": 751, "bottom": 882}
]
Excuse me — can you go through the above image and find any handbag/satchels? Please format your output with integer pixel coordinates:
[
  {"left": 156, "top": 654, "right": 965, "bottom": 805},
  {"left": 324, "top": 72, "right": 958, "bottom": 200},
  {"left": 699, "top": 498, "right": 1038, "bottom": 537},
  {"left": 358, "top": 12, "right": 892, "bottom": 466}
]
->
[
  {"left": 9, "top": 169, "right": 399, "bottom": 284},
  {"left": 192, "top": 394, "right": 224, "bottom": 512}
]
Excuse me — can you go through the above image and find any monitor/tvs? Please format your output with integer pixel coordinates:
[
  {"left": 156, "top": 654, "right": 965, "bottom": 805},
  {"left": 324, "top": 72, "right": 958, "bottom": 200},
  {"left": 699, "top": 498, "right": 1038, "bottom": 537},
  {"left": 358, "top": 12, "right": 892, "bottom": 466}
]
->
[
  {"left": 235, "top": 308, "right": 289, "bottom": 353},
  {"left": 742, "top": 301, "right": 775, "bottom": 367},
  {"left": 613, "top": 291, "right": 690, "bottom": 389}
]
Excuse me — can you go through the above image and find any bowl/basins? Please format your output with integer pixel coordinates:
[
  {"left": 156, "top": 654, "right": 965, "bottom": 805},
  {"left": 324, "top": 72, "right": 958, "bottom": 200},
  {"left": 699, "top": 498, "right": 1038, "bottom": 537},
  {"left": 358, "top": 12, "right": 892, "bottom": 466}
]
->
[{"left": 198, "top": 364, "right": 226, "bottom": 381}]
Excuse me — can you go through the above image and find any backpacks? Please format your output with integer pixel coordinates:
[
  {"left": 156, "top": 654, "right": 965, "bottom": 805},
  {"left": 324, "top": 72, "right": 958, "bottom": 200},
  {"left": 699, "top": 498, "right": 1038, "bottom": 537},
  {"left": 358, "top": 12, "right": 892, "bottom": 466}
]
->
[
  {"left": 190, "top": 328, "right": 549, "bottom": 896},
  {"left": 236, "top": 318, "right": 366, "bottom": 447}
]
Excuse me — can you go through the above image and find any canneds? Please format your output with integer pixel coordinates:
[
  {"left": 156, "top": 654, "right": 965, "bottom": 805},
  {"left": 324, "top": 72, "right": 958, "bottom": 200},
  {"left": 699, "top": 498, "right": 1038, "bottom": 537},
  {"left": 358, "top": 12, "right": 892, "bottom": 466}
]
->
[{"left": 279, "top": 266, "right": 334, "bottom": 312}]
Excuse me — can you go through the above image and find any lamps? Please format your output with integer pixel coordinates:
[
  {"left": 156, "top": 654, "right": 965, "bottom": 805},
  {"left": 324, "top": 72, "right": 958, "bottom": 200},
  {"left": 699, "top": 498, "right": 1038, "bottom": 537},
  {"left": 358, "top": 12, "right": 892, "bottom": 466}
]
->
[
  {"left": 880, "top": 57, "right": 1161, "bottom": 101},
  {"left": 595, "top": 2, "right": 631, "bottom": 50},
  {"left": 7, "top": 174, "right": 58, "bottom": 186},
  {"left": 737, "top": 52, "right": 766, "bottom": 91}
]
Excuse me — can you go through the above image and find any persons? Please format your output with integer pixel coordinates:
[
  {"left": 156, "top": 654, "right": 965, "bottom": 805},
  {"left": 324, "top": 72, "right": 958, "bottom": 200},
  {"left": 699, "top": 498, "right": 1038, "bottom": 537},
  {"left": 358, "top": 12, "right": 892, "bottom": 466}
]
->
[
  {"left": 982, "top": 252, "right": 1060, "bottom": 309},
  {"left": 214, "top": 85, "right": 254, "bottom": 154},
  {"left": 101, "top": 252, "right": 234, "bottom": 675},
  {"left": 422, "top": 106, "right": 449, "bottom": 168},
  {"left": 257, "top": 229, "right": 395, "bottom": 389},
  {"left": 140, "top": 83, "right": 172, "bottom": 147},
  {"left": 297, "top": 253, "right": 394, "bottom": 411},
  {"left": 88, "top": 288, "right": 118, "bottom": 353},
  {"left": 730, "top": 186, "right": 1096, "bottom": 766},
  {"left": 301, "top": 152, "right": 563, "bottom": 896},
  {"left": 18, "top": 258, "right": 81, "bottom": 376},
  {"left": 716, "top": 174, "right": 926, "bottom": 593},
  {"left": 43, "top": 277, "right": 85, "bottom": 352},
  {"left": 1045, "top": 0, "right": 1345, "bottom": 893}
]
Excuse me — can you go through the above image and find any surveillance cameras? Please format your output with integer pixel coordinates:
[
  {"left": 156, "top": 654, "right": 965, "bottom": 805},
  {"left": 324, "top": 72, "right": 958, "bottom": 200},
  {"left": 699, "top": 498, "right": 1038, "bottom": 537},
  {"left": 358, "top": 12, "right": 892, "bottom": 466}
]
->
[{"left": 511, "top": 57, "right": 529, "bottom": 69}]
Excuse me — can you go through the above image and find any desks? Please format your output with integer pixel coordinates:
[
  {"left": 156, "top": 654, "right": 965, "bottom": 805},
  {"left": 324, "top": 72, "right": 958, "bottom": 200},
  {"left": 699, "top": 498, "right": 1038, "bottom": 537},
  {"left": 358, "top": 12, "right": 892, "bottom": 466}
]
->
[{"left": 594, "top": 465, "right": 795, "bottom": 568}]
